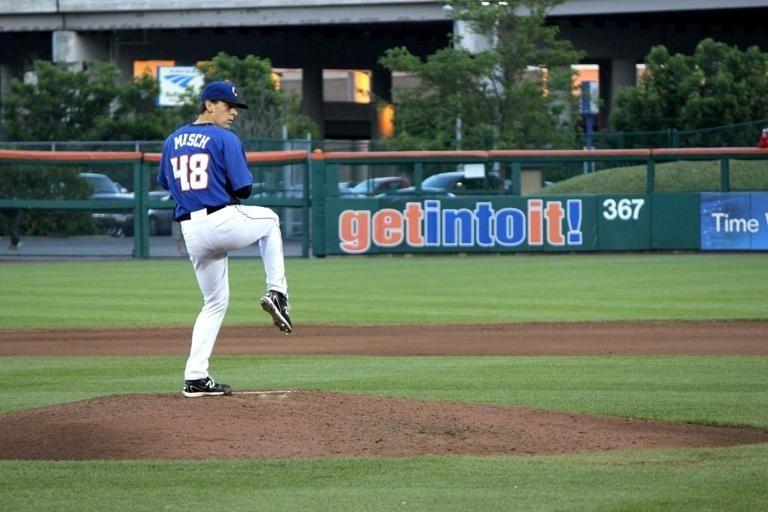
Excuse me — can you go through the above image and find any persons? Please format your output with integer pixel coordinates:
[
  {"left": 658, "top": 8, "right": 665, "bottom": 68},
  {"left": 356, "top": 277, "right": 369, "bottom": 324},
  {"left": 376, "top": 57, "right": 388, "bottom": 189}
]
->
[{"left": 156, "top": 81, "right": 294, "bottom": 398}]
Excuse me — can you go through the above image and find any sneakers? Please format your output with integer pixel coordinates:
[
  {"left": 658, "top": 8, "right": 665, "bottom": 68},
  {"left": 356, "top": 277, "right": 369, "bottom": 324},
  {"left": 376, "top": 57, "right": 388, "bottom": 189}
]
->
[
  {"left": 258, "top": 290, "right": 294, "bottom": 335},
  {"left": 182, "top": 376, "right": 233, "bottom": 398}
]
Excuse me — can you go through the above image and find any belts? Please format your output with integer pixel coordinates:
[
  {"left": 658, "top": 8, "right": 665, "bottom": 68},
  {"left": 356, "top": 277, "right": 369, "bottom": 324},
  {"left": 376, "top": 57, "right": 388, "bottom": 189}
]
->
[{"left": 178, "top": 204, "right": 227, "bottom": 222}]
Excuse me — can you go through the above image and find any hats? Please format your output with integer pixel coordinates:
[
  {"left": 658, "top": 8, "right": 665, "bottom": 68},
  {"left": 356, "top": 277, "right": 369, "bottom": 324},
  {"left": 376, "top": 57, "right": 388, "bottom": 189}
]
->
[{"left": 200, "top": 81, "right": 249, "bottom": 110}]
